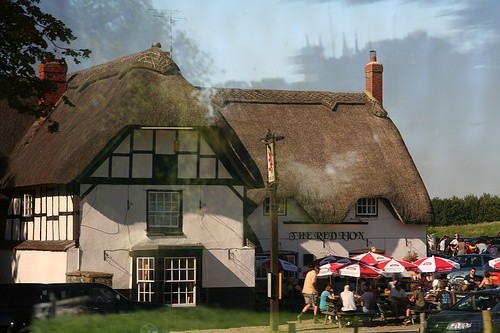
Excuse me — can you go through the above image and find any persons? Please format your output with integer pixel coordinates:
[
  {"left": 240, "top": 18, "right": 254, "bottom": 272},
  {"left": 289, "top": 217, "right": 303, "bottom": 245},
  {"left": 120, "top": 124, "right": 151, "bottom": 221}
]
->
[
  {"left": 298, "top": 268, "right": 323, "bottom": 325},
  {"left": 319, "top": 285, "right": 339, "bottom": 321},
  {"left": 426, "top": 233, "right": 496, "bottom": 259},
  {"left": 340, "top": 285, "right": 357, "bottom": 325},
  {"left": 358, "top": 269, "right": 493, "bottom": 326}
]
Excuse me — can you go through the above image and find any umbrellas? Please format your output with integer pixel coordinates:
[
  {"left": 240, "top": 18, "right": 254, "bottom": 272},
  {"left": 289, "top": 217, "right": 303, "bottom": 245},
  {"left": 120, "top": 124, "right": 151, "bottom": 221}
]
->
[
  {"left": 317, "top": 249, "right": 460, "bottom": 295},
  {"left": 488, "top": 257, "right": 500, "bottom": 271},
  {"left": 254, "top": 259, "right": 301, "bottom": 272}
]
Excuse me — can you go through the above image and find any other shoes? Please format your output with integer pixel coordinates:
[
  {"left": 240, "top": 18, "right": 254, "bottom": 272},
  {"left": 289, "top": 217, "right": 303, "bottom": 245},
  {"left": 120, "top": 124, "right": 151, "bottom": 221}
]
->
[
  {"left": 297, "top": 313, "right": 302, "bottom": 324},
  {"left": 314, "top": 316, "right": 322, "bottom": 324},
  {"left": 402, "top": 319, "right": 411, "bottom": 324}
]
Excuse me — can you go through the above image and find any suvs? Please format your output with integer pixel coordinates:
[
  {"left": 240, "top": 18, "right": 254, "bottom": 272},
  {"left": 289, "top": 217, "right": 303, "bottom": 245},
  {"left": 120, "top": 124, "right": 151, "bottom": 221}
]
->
[{"left": 0, "top": 282, "right": 168, "bottom": 333}]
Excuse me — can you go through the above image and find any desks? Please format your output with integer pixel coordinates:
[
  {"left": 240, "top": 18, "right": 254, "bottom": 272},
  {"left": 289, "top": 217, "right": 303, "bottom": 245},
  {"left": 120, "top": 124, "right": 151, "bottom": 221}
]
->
[{"left": 450, "top": 289, "right": 500, "bottom": 312}]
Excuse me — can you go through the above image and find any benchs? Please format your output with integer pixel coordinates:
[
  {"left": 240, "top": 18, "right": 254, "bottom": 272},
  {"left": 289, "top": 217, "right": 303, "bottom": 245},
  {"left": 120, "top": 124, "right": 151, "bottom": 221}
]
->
[
  {"left": 322, "top": 311, "right": 392, "bottom": 328},
  {"left": 410, "top": 310, "right": 442, "bottom": 326}
]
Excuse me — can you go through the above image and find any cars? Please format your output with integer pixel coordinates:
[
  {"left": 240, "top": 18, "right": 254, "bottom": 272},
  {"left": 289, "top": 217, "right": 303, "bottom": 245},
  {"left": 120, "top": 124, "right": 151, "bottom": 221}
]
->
[
  {"left": 424, "top": 289, "right": 500, "bottom": 333},
  {"left": 433, "top": 234, "right": 500, "bottom": 288}
]
